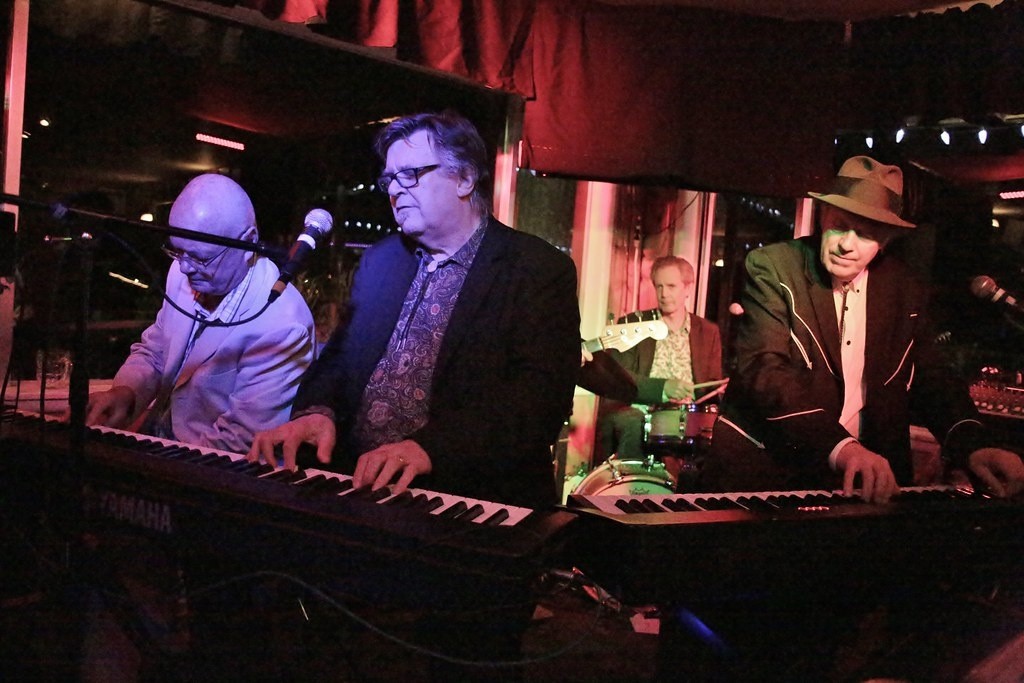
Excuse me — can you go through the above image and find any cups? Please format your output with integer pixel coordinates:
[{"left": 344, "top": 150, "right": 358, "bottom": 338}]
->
[{"left": 37, "top": 348, "right": 71, "bottom": 389}]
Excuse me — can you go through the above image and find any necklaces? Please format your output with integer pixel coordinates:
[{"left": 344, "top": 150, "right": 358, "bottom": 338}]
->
[{"left": 394, "top": 257, "right": 439, "bottom": 353}]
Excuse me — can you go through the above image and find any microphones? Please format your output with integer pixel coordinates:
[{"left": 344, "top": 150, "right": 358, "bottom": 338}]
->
[
  {"left": 971, "top": 275, "right": 1024, "bottom": 326},
  {"left": 268, "top": 209, "right": 333, "bottom": 303}
]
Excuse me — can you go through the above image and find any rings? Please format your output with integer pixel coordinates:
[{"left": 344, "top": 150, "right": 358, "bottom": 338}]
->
[{"left": 394, "top": 454, "right": 404, "bottom": 463}]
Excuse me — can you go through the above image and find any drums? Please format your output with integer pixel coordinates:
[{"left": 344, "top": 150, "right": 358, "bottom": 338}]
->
[
  {"left": 643, "top": 400, "right": 718, "bottom": 447},
  {"left": 574, "top": 459, "right": 683, "bottom": 495}
]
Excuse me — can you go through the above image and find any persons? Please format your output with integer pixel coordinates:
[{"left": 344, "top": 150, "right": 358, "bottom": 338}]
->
[
  {"left": 580, "top": 339, "right": 594, "bottom": 368},
  {"left": 80, "top": 171, "right": 328, "bottom": 668},
  {"left": 598, "top": 256, "right": 722, "bottom": 485},
  {"left": 700, "top": 155, "right": 1024, "bottom": 683},
  {"left": 245, "top": 111, "right": 582, "bottom": 683}
]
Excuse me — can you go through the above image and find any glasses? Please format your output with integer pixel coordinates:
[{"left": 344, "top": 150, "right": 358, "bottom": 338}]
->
[
  {"left": 161, "top": 226, "right": 251, "bottom": 272},
  {"left": 378, "top": 163, "right": 441, "bottom": 194}
]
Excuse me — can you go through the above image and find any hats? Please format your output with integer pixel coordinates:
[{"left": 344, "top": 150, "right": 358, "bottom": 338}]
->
[{"left": 807, "top": 157, "right": 914, "bottom": 228}]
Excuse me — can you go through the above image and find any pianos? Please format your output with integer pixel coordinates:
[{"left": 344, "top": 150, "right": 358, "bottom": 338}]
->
[
  {"left": 0, "top": 416, "right": 577, "bottom": 568},
  {"left": 559, "top": 479, "right": 1024, "bottom": 605}
]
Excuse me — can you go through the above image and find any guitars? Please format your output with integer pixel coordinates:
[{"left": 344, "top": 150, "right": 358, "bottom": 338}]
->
[
  {"left": 969, "top": 379, "right": 1024, "bottom": 419},
  {"left": 580, "top": 311, "right": 668, "bottom": 367}
]
